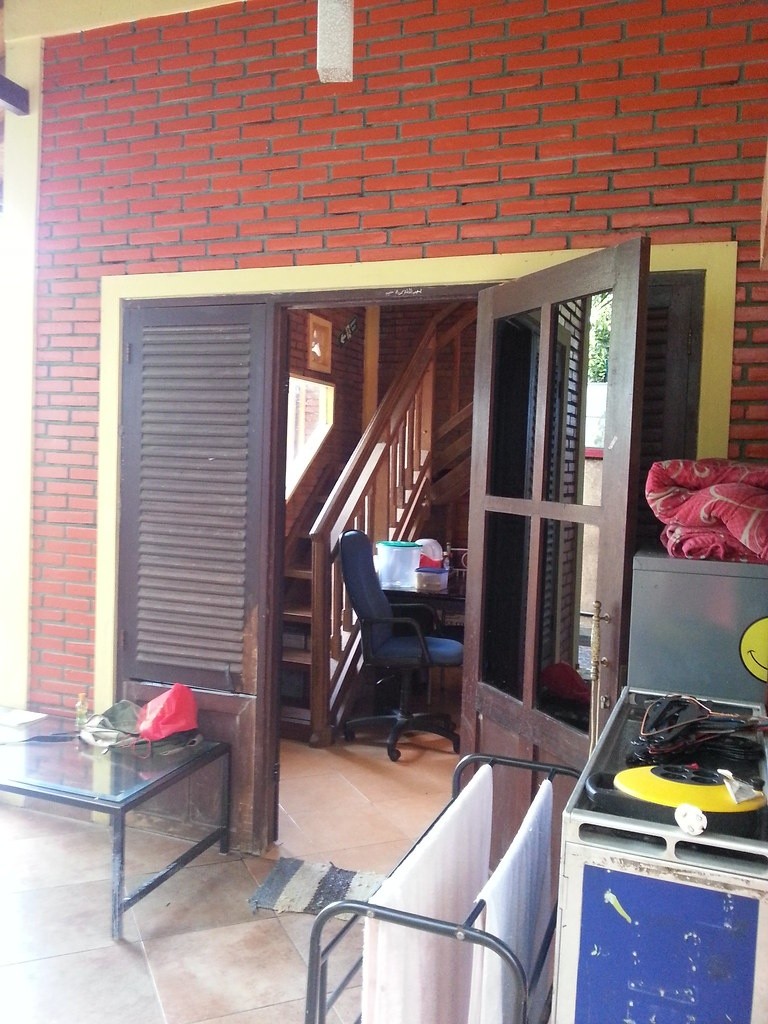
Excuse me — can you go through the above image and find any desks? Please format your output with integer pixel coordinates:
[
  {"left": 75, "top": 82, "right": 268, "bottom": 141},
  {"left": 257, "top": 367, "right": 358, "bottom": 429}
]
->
[
  {"left": 0, "top": 704, "right": 231, "bottom": 943},
  {"left": 379, "top": 582, "right": 466, "bottom": 706}
]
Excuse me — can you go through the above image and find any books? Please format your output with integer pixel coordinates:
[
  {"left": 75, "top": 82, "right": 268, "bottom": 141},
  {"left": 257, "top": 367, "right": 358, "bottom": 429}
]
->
[{"left": 1, "top": 709, "right": 48, "bottom": 729}]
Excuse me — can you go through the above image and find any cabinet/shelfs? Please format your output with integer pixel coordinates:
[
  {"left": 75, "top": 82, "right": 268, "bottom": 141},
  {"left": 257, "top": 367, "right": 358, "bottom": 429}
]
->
[{"left": 304, "top": 752, "right": 583, "bottom": 1023}]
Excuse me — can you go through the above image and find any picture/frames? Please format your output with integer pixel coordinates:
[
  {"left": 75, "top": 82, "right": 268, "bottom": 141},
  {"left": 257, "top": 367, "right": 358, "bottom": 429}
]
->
[{"left": 305, "top": 312, "right": 332, "bottom": 374}]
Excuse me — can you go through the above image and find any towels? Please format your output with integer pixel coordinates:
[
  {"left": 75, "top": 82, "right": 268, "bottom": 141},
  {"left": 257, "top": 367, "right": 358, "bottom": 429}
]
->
[
  {"left": 465, "top": 777, "right": 554, "bottom": 1023},
  {"left": 360, "top": 763, "right": 493, "bottom": 1024}
]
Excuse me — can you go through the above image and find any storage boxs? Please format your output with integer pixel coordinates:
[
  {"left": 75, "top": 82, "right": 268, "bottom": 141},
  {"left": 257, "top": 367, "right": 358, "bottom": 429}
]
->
[{"left": 375, "top": 540, "right": 423, "bottom": 588}]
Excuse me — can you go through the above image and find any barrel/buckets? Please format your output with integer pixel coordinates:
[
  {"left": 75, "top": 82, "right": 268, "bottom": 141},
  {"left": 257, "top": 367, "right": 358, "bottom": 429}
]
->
[{"left": 376, "top": 541, "right": 422, "bottom": 590}]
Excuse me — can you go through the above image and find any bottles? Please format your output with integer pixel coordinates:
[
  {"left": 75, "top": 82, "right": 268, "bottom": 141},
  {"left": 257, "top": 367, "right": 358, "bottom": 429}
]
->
[{"left": 76, "top": 693, "right": 87, "bottom": 730}]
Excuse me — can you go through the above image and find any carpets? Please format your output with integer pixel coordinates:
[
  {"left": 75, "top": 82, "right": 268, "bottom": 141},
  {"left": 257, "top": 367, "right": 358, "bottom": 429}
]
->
[{"left": 246, "top": 856, "right": 388, "bottom": 924}]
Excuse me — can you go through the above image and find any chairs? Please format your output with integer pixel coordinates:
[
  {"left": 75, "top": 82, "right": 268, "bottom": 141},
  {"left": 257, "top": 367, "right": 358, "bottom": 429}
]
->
[{"left": 338, "top": 529, "right": 460, "bottom": 761}]
